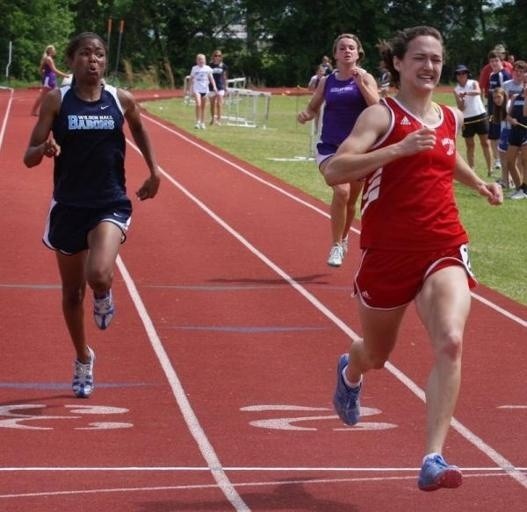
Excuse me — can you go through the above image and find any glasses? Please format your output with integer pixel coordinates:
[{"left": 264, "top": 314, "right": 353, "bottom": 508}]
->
[{"left": 214, "top": 55, "right": 221, "bottom": 57}]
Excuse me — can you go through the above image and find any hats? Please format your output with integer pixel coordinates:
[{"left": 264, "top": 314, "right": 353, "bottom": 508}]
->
[
  {"left": 455, "top": 65, "right": 469, "bottom": 76},
  {"left": 491, "top": 44, "right": 506, "bottom": 53}
]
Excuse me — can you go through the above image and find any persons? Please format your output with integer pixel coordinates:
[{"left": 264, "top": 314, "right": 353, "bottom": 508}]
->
[
  {"left": 207, "top": 50, "right": 229, "bottom": 126},
  {"left": 31, "top": 45, "right": 69, "bottom": 115},
  {"left": 298, "top": 34, "right": 379, "bottom": 266},
  {"left": 187, "top": 53, "right": 217, "bottom": 130},
  {"left": 321, "top": 26, "right": 504, "bottom": 491},
  {"left": 23, "top": 31, "right": 161, "bottom": 398},
  {"left": 452, "top": 43, "right": 526, "bottom": 199}
]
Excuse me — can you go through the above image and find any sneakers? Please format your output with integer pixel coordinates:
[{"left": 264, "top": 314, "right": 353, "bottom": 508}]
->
[
  {"left": 495, "top": 159, "right": 527, "bottom": 199},
  {"left": 328, "top": 246, "right": 344, "bottom": 265},
  {"left": 195, "top": 119, "right": 222, "bottom": 129},
  {"left": 342, "top": 234, "right": 348, "bottom": 253},
  {"left": 418, "top": 455, "right": 463, "bottom": 491},
  {"left": 334, "top": 354, "right": 362, "bottom": 425},
  {"left": 93, "top": 288, "right": 113, "bottom": 330},
  {"left": 72, "top": 345, "right": 94, "bottom": 398}
]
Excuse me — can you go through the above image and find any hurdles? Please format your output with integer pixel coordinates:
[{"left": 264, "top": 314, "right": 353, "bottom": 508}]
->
[
  {"left": 268, "top": 92, "right": 320, "bottom": 165},
  {"left": 184, "top": 76, "right": 273, "bottom": 129}
]
